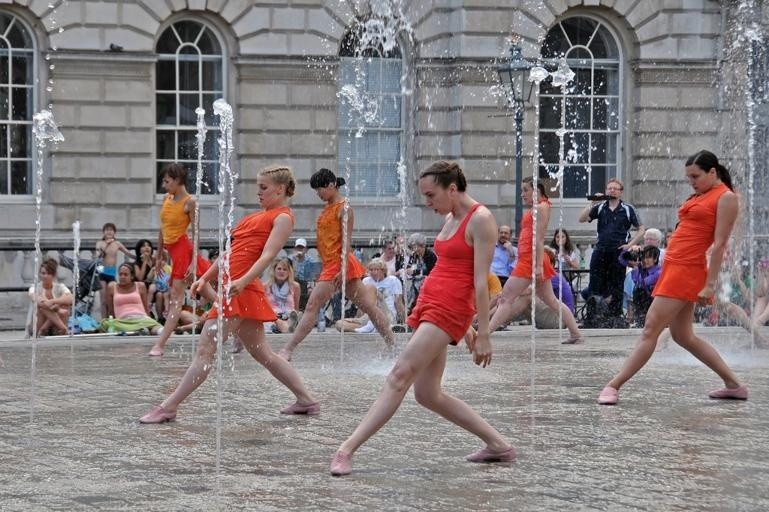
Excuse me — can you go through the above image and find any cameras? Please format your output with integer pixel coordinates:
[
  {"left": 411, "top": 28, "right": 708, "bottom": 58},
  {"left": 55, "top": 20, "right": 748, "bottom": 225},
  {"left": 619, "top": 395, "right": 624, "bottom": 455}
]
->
[{"left": 622, "top": 248, "right": 644, "bottom": 262}]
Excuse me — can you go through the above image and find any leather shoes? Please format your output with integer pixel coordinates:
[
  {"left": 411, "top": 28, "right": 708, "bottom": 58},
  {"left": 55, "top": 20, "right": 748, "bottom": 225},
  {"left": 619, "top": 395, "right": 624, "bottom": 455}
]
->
[
  {"left": 597, "top": 386, "right": 619, "bottom": 405},
  {"left": 561, "top": 336, "right": 584, "bottom": 345},
  {"left": 465, "top": 444, "right": 518, "bottom": 464},
  {"left": 329, "top": 448, "right": 354, "bottom": 475},
  {"left": 279, "top": 400, "right": 321, "bottom": 416},
  {"left": 139, "top": 405, "right": 177, "bottom": 425},
  {"left": 229, "top": 339, "right": 293, "bottom": 363},
  {"left": 148, "top": 344, "right": 165, "bottom": 357},
  {"left": 708, "top": 384, "right": 749, "bottom": 401}
]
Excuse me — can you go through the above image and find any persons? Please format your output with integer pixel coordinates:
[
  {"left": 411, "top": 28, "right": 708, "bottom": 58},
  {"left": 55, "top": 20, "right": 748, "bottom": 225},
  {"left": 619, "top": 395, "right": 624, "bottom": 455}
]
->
[
  {"left": 28, "top": 223, "right": 232, "bottom": 338},
  {"left": 275, "top": 167, "right": 398, "bottom": 361},
  {"left": 595, "top": 150, "right": 749, "bottom": 404},
  {"left": 260, "top": 233, "right": 435, "bottom": 334},
  {"left": 487, "top": 175, "right": 582, "bottom": 343},
  {"left": 487, "top": 224, "right": 768, "bottom": 347},
  {"left": 331, "top": 162, "right": 518, "bottom": 475},
  {"left": 140, "top": 161, "right": 318, "bottom": 425},
  {"left": 148, "top": 162, "right": 245, "bottom": 356},
  {"left": 578, "top": 180, "right": 642, "bottom": 313}
]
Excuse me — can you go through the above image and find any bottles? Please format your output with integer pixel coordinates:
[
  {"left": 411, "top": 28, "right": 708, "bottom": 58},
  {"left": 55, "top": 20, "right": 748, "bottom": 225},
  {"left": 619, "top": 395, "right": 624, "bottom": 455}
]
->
[
  {"left": 106, "top": 315, "right": 116, "bottom": 332},
  {"left": 68, "top": 315, "right": 79, "bottom": 333},
  {"left": 318, "top": 308, "right": 326, "bottom": 331}
]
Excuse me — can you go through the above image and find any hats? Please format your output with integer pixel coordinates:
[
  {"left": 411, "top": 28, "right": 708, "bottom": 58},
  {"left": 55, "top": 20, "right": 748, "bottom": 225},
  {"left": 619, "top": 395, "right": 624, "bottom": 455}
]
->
[{"left": 294, "top": 238, "right": 308, "bottom": 248}]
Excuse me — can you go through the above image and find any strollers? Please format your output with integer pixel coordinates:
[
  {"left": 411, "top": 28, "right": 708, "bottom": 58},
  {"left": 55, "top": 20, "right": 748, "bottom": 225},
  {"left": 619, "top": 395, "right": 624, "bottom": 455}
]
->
[{"left": 57, "top": 255, "right": 102, "bottom": 301}]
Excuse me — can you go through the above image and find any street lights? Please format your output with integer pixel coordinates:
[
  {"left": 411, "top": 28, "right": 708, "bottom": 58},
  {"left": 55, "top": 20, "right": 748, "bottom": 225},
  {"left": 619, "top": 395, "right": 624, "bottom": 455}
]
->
[{"left": 496, "top": 41, "right": 538, "bottom": 239}]
